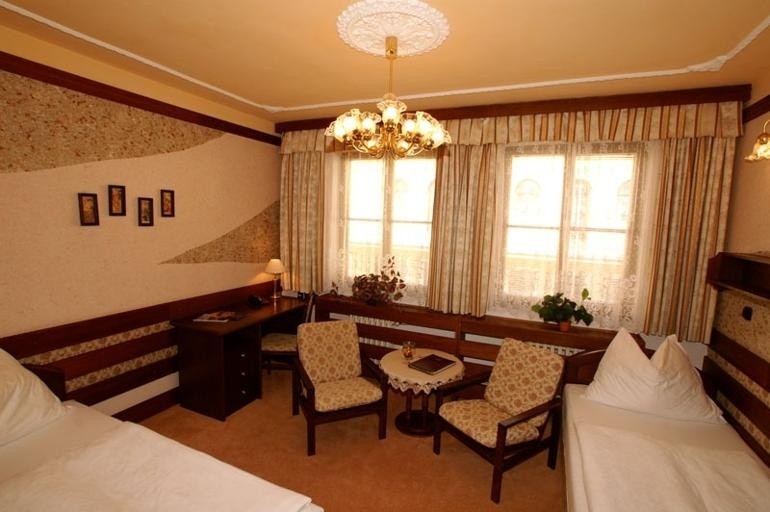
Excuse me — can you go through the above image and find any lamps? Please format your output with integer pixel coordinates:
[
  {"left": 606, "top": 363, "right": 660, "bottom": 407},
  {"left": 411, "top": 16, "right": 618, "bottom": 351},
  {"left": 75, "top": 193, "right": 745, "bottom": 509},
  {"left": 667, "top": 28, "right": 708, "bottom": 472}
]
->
[
  {"left": 743, "top": 118, "right": 770, "bottom": 162},
  {"left": 264, "top": 259, "right": 285, "bottom": 299},
  {"left": 321, "top": 94, "right": 451, "bottom": 160}
]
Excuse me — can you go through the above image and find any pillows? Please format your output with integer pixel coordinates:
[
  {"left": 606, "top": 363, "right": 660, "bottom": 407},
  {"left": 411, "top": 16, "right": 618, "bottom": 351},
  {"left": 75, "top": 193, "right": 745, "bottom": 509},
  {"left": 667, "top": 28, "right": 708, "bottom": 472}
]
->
[
  {"left": 1, "top": 347, "right": 76, "bottom": 446},
  {"left": 579, "top": 326, "right": 729, "bottom": 427}
]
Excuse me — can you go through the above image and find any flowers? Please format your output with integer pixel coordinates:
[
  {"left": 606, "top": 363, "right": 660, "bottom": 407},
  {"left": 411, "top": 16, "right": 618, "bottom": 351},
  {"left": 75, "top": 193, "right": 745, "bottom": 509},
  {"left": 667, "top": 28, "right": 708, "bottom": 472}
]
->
[{"left": 351, "top": 257, "right": 406, "bottom": 306}]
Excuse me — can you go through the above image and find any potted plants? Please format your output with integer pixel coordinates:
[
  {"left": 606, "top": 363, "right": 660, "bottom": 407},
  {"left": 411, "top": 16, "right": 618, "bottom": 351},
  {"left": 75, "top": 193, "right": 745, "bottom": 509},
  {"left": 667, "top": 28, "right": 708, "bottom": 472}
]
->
[{"left": 530, "top": 289, "right": 595, "bottom": 332}]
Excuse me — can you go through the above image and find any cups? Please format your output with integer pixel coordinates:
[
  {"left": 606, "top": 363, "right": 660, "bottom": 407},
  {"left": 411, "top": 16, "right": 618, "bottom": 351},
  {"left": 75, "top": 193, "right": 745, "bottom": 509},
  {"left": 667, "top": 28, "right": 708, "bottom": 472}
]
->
[{"left": 400, "top": 340, "right": 415, "bottom": 360}]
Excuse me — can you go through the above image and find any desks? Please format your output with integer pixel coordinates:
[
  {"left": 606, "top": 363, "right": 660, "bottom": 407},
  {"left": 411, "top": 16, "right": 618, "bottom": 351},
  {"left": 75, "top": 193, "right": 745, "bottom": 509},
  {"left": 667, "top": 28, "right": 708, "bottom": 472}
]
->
[{"left": 170, "top": 294, "right": 307, "bottom": 421}]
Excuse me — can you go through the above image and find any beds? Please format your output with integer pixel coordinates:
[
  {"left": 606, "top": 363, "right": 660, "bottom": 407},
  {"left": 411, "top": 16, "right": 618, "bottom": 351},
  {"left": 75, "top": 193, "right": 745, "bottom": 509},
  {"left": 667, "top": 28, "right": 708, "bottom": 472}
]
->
[
  {"left": 564, "top": 347, "right": 770, "bottom": 512},
  {"left": 1, "top": 345, "right": 318, "bottom": 510}
]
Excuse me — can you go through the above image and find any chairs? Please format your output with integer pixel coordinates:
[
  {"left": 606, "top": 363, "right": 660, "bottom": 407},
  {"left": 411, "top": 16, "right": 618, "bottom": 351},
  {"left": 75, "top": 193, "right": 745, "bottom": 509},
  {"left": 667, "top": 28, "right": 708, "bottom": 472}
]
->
[
  {"left": 434, "top": 335, "right": 561, "bottom": 502},
  {"left": 275, "top": 292, "right": 315, "bottom": 330},
  {"left": 261, "top": 306, "right": 319, "bottom": 374},
  {"left": 291, "top": 318, "right": 389, "bottom": 455}
]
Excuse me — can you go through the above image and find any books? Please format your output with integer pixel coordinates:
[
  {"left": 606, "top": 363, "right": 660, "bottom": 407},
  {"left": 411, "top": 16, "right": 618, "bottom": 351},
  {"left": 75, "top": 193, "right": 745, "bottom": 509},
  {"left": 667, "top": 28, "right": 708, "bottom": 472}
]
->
[{"left": 408, "top": 354, "right": 457, "bottom": 376}]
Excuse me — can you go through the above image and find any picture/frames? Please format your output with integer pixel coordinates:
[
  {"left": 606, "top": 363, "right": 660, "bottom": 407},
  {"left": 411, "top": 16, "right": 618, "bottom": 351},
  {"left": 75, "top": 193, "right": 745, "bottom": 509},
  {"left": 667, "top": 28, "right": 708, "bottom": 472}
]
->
[
  {"left": 138, "top": 196, "right": 154, "bottom": 226},
  {"left": 108, "top": 184, "right": 124, "bottom": 215},
  {"left": 78, "top": 191, "right": 100, "bottom": 225},
  {"left": 160, "top": 188, "right": 174, "bottom": 217}
]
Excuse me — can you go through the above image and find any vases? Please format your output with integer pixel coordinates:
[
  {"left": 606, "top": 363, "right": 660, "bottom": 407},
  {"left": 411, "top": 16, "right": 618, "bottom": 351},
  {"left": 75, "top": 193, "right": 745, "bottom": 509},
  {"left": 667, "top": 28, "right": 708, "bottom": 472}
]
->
[{"left": 367, "top": 298, "right": 381, "bottom": 307}]
